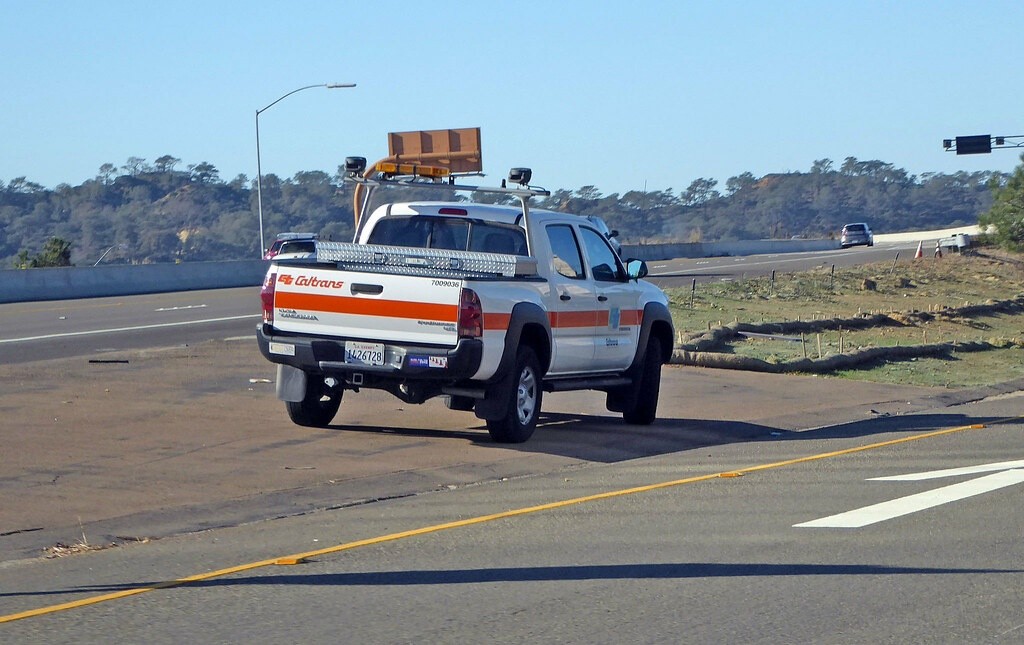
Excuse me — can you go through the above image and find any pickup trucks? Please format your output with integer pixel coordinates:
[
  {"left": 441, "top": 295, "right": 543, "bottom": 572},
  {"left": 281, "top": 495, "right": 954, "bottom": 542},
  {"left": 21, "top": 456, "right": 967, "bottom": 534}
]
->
[{"left": 256, "top": 200, "right": 675, "bottom": 442}]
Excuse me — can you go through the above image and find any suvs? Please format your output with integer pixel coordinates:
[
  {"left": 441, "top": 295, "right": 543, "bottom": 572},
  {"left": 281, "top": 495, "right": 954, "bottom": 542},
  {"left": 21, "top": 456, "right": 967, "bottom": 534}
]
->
[{"left": 263, "top": 234, "right": 318, "bottom": 261}]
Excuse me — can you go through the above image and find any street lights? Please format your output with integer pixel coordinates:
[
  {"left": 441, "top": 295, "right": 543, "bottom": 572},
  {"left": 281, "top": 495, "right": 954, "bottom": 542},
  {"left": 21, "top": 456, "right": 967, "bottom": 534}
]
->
[
  {"left": 256, "top": 80, "right": 357, "bottom": 260},
  {"left": 94, "top": 244, "right": 123, "bottom": 266}
]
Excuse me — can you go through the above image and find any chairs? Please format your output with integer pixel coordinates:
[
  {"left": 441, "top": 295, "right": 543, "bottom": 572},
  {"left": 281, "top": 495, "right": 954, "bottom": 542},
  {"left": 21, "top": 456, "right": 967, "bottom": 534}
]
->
[
  {"left": 388, "top": 216, "right": 456, "bottom": 251},
  {"left": 481, "top": 234, "right": 515, "bottom": 255}
]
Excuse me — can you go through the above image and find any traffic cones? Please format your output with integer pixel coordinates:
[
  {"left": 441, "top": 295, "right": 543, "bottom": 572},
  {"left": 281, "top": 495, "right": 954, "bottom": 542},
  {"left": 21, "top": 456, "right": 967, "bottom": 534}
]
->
[
  {"left": 934, "top": 241, "right": 943, "bottom": 258},
  {"left": 913, "top": 241, "right": 923, "bottom": 259}
]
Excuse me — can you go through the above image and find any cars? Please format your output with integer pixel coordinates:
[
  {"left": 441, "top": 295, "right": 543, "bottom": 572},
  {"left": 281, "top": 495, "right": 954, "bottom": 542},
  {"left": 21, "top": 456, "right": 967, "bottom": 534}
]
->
[
  {"left": 271, "top": 238, "right": 320, "bottom": 260},
  {"left": 840, "top": 223, "right": 874, "bottom": 250}
]
